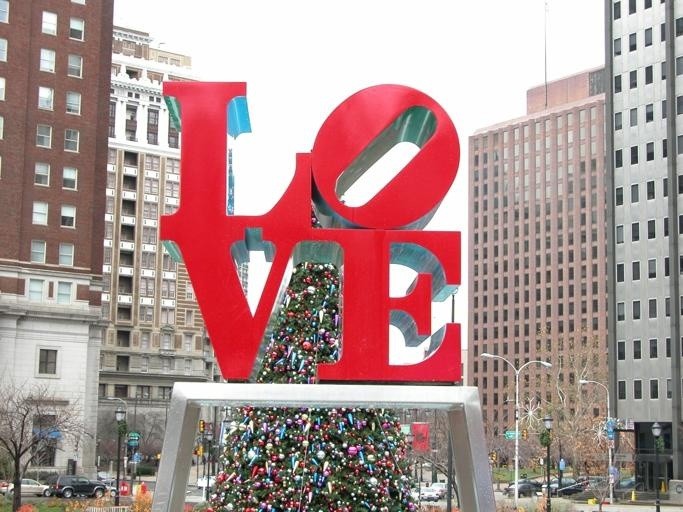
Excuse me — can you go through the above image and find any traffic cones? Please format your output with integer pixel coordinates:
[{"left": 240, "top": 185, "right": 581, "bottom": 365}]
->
[
  {"left": 661, "top": 480, "right": 665, "bottom": 492},
  {"left": 631, "top": 491, "right": 636, "bottom": 501}
]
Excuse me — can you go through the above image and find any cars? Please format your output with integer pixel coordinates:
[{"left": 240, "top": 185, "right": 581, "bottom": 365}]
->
[
  {"left": 504, "top": 476, "right": 603, "bottom": 498},
  {"left": 0, "top": 471, "right": 116, "bottom": 498},
  {"left": 411, "top": 482, "right": 455, "bottom": 502}
]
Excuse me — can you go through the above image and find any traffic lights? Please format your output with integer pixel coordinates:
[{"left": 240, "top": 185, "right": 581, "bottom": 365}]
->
[
  {"left": 199, "top": 419, "right": 205, "bottom": 433},
  {"left": 194, "top": 450, "right": 200, "bottom": 455}
]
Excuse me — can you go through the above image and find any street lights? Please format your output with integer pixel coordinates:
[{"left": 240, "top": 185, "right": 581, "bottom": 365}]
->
[
  {"left": 580, "top": 380, "right": 613, "bottom": 505},
  {"left": 652, "top": 421, "right": 661, "bottom": 512},
  {"left": 205, "top": 431, "right": 213, "bottom": 501},
  {"left": 107, "top": 397, "right": 128, "bottom": 507},
  {"left": 542, "top": 413, "right": 554, "bottom": 511},
  {"left": 481, "top": 353, "right": 552, "bottom": 512}
]
